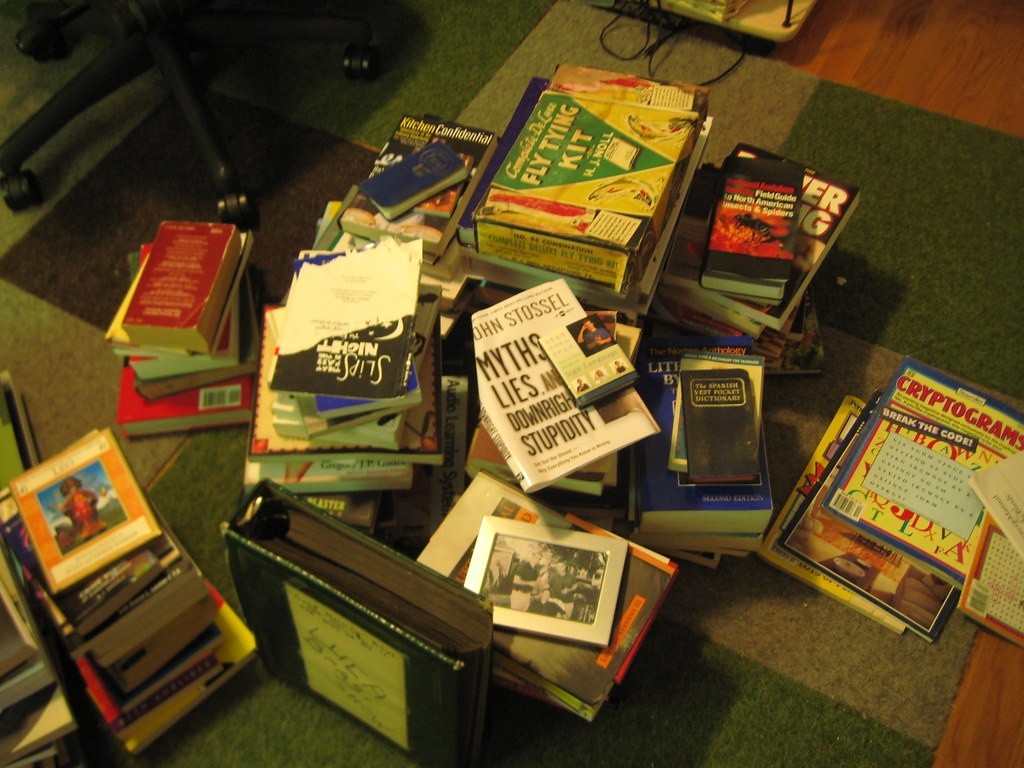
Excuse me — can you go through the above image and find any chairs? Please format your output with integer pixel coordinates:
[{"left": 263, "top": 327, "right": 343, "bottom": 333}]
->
[{"left": 0, "top": 0, "right": 376, "bottom": 225}]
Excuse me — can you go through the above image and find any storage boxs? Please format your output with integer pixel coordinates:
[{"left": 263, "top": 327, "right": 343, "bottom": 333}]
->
[{"left": 471, "top": 63, "right": 711, "bottom": 293}]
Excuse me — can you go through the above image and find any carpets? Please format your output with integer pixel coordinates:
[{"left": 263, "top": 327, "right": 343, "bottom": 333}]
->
[{"left": 0, "top": 0, "right": 1024, "bottom": 768}]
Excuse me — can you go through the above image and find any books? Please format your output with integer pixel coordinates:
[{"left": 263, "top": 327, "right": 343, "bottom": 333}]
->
[{"left": 0, "top": 62, "right": 1024, "bottom": 768}]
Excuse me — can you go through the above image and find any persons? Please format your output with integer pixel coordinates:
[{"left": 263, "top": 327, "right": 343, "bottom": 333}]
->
[
  {"left": 509, "top": 551, "right": 541, "bottom": 611},
  {"left": 594, "top": 370, "right": 607, "bottom": 384},
  {"left": 576, "top": 380, "right": 589, "bottom": 393},
  {"left": 541, "top": 560, "right": 577, "bottom": 620},
  {"left": 577, "top": 319, "right": 614, "bottom": 354},
  {"left": 614, "top": 361, "right": 627, "bottom": 374}
]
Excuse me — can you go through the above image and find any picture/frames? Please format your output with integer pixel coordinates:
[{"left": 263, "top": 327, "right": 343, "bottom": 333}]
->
[{"left": 463, "top": 513, "right": 628, "bottom": 647}]
